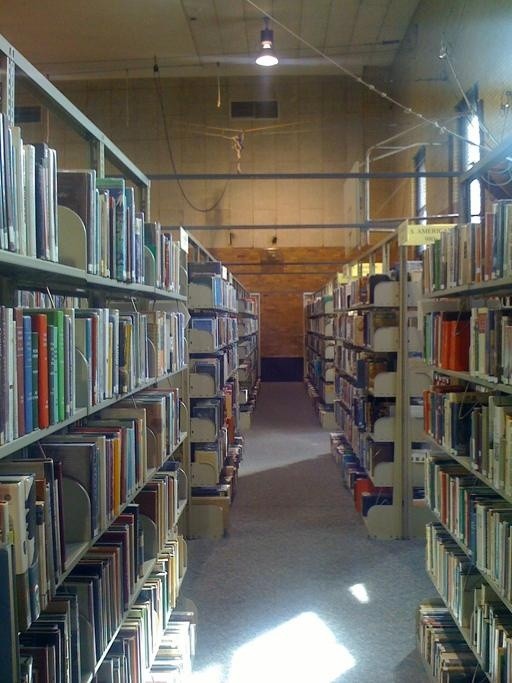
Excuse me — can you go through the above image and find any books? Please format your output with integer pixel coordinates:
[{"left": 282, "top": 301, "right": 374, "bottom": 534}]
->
[
  {"left": 0, "top": 113, "right": 198, "bottom": 683},
  {"left": 187, "top": 241, "right": 261, "bottom": 531},
  {"left": 407, "top": 199, "right": 511, "bottom": 683},
  {"left": 304, "top": 265, "right": 400, "bottom": 521}
]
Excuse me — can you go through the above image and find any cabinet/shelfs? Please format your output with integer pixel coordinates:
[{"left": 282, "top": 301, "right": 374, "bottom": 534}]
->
[
  {"left": 1, "top": 29, "right": 192, "bottom": 682},
  {"left": 299, "top": 222, "right": 432, "bottom": 543},
  {"left": 174, "top": 232, "right": 261, "bottom": 540},
  {"left": 417, "top": 199, "right": 512, "bottom": 680}
]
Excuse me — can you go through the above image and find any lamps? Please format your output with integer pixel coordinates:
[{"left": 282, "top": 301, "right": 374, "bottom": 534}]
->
[{"left": 255, "top": 14, "right": 280, "bottom": 67}]
